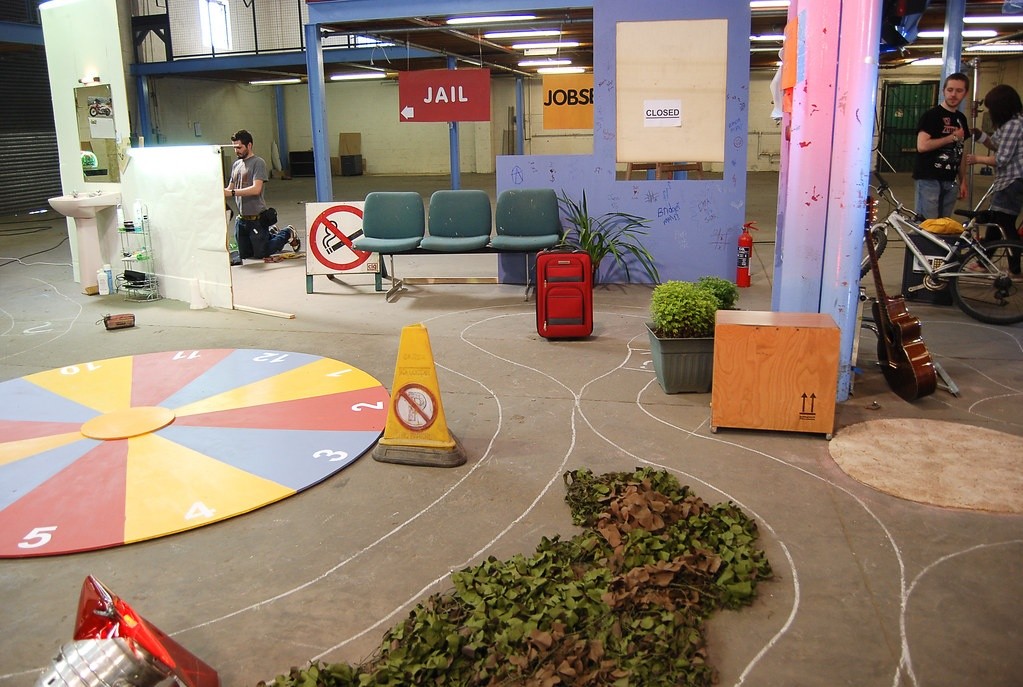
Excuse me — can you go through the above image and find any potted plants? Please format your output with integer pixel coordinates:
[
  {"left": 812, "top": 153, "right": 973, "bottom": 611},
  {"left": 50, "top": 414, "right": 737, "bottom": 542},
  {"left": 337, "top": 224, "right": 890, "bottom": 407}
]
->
[
  {"left": 529, "top": 199, "right": 660, "bottom": 288},
  {"left": 644, "top": 278, "right": 721, "bottom": 393}
]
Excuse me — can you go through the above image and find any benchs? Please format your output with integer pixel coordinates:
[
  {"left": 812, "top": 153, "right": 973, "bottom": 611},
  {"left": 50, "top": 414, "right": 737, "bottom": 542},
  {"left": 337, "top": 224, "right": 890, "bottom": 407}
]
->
[{"left": 354, "top": 188, "right": 567, "bottom": 297}]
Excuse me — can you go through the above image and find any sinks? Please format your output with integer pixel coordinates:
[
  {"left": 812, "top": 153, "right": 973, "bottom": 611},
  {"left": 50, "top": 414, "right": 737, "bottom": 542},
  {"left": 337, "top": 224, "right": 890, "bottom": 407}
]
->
[{"left": 47, "top": 193, "right": 121, "bottom": 215}]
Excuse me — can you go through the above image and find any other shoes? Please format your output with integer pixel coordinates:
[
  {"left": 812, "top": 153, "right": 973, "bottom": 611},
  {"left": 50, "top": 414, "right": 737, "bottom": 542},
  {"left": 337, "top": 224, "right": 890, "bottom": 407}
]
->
[
  {"left": 964, "top": 260, "right": 987, "bottom": 278},
  {"left": 993, "top": 269, "right": 1023, "bottom": 283}
]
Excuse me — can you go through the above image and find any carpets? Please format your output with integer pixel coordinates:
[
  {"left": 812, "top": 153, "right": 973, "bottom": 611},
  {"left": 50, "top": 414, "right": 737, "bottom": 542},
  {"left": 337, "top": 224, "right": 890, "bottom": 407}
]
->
[{"left": 828, "top": 418, "right": 1022, "bottom": 516}]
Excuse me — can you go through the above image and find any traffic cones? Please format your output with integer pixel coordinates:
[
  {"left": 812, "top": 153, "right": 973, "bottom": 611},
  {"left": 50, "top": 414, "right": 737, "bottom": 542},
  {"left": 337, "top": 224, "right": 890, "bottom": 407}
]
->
[{"left": 368, "top": 322, "right": 467, "bottom": 470}]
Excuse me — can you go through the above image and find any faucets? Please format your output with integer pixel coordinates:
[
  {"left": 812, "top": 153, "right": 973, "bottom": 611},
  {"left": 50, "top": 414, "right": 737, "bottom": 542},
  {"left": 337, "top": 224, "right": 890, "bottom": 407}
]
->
[{"left": 71, "top": 189, "right": 102, "bottom": 199}]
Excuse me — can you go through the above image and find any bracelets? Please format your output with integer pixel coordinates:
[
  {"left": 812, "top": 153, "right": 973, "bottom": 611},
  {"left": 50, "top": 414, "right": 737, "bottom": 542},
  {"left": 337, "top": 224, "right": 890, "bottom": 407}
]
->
[{"left": 977, "top": 133, "right": 987, "bottom": 145}]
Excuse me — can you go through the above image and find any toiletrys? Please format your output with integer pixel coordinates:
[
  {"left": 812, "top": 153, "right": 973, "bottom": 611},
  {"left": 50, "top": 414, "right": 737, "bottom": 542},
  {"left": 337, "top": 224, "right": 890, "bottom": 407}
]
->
[
  {"left": 116, "top": 200, "right": 143, "bottom": 234},
  {"left": 96, "top": 264, "right": 112, "bottom": 296}
]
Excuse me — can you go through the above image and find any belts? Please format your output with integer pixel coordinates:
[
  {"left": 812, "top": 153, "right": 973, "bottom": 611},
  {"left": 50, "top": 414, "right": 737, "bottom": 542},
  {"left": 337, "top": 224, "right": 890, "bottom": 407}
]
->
[{"left": 239, "top": 215, "right": 257, "bottom": 221}]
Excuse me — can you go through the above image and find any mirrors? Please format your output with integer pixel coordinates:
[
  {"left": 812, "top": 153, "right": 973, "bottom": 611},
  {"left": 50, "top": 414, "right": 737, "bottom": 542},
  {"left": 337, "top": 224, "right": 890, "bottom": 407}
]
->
[{"left": 74, "top": 84, "right": 121, "bottom": 184}]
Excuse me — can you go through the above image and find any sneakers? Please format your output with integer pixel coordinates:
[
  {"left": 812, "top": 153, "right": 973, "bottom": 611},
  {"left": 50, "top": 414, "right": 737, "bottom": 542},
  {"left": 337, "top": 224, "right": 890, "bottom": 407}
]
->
[
  {"left": 268, "top": 225, "right": 280, "bottom": 252},
  {"left": 286, "top": 224, "right": 301, "bottom": 253}
]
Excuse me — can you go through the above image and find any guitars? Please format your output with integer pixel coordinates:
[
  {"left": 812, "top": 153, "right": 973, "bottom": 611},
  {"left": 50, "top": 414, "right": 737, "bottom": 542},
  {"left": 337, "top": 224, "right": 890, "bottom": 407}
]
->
[{"left": 863, "top": 195, "right": 938, "bottom": 403}]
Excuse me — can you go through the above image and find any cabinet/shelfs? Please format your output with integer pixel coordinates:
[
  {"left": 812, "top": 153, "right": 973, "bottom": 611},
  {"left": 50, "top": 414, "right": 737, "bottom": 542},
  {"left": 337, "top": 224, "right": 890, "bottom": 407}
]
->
[
  {"left": 116, "top": 201, "right": 161, "bottom": 302},
  {"left": 711, "top": 311, "right": 841, "bottom": 439}
]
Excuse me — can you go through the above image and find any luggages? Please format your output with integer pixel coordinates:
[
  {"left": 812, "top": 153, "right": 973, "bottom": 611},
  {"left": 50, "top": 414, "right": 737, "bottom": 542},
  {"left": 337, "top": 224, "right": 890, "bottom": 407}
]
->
[{"left": 536, "top": 243, "right": 593, "bottom": 341}]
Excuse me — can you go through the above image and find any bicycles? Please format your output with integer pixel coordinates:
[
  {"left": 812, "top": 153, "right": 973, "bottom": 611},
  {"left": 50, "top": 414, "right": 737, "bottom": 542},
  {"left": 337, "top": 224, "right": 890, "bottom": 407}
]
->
[{"left": 856, "top": 168, "right": 1023, "bottom": 325}]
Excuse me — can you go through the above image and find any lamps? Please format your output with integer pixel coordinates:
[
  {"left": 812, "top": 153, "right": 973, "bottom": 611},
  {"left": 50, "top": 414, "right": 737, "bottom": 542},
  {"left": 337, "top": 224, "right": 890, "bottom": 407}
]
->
[
  {"left": 78, "top": 76, "right": 100, "bottom": 84},
  {"left": 248, "top": 4, "right": 1023, "bottom": 88}
]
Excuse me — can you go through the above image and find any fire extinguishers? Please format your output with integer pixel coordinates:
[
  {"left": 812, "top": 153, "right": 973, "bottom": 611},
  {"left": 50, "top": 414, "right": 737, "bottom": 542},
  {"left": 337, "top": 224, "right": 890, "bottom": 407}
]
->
[{"left": 736, "top": 221, "right": 760, "bottom": 287}]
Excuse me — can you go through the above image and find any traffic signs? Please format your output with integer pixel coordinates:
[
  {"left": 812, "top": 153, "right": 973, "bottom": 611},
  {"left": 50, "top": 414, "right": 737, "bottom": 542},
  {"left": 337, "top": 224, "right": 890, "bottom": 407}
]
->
[{"left": 397, "top": 67, "right": 491, "bottom": 122}]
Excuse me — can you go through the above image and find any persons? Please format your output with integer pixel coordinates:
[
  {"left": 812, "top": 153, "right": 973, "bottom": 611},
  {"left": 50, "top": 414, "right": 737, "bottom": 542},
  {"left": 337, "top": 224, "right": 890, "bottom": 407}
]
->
[
  {"left": 966, "top": 84, "right": 1023, "bottom": 283},
  {"left": 913, "top": 73, "right": 971, "bottom": 224},
  {"left": 224, "top": 130, "right": 301, "bottom": 260}
]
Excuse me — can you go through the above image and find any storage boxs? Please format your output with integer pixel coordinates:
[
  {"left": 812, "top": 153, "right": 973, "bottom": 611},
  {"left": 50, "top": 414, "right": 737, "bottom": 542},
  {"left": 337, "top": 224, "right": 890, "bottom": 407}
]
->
[{"left": 287, "top": 132, "right": 366, "bottom": 178}]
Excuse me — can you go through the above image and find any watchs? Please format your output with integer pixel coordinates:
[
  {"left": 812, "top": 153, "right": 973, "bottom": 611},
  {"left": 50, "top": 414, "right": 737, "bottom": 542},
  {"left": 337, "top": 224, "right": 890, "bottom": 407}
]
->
[
  {"left": 231, "top": 190, "right": 236, "bottom": 197},
  {"left": 952, "top": 134, "right": 959, "bottom": 143}
]
